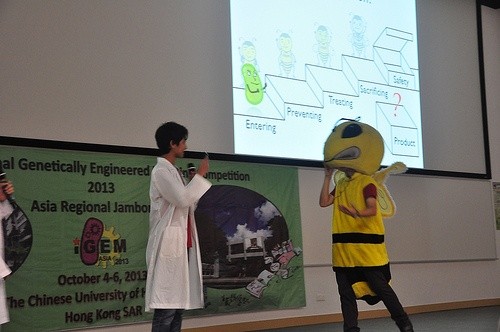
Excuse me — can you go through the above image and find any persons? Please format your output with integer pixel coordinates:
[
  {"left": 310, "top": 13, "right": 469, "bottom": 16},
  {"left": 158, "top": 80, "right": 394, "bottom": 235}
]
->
[
  {"left": 0, "top": 179, "right": 16, "bottom": 327},
  {"left": 145, "top": 122, "right": 213, "bottom": 332},
  {"left": 320, "top": 121, "right": 416, "bottom": 332}
]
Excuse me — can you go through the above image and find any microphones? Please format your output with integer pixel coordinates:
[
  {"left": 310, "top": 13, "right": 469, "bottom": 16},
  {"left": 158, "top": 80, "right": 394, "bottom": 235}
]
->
[
  {"left": 188, "top": 163, "right": 196, "bottom": 174},
  {"left": 0, "top": 166, "right": 16, "bottom": 208}
]
